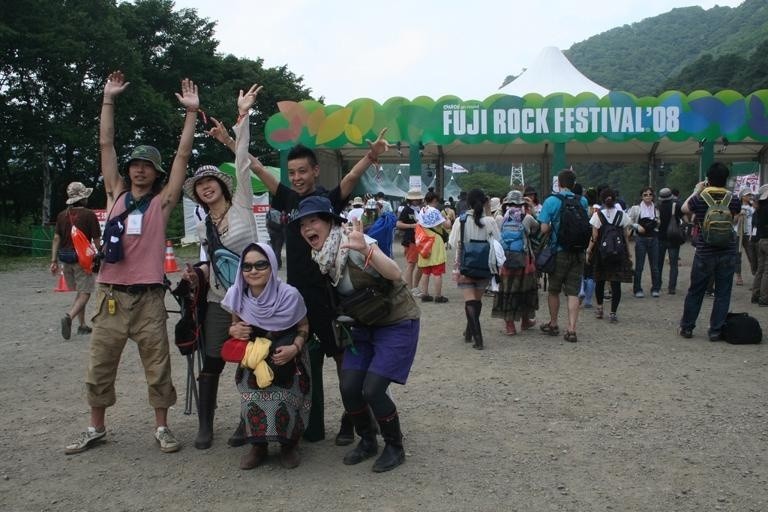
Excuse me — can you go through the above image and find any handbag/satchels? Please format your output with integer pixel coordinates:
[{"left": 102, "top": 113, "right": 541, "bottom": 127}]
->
[
  {"left": 336, "top": 283, "right": 394, "bottom": 325},
  {"left": 206, "top": 242, "right": 241, "bottom": 296},
  {"left": 666, "top": 214, "right": 687, "bottom": 247},
  {"left": 536, "top": 244, "right": 558, "bottom": 275},
  {"left": 329, "top": 318, "right": 361, "bottom": 350},
  {"left": 719, "top": 309, "right": 764, "bottom": 348},
  {"left": 455, "top": 237, "right": 496, "bottom": 284},
  {"left": 448, "top": 218, "right": 453, "bottom": 229},
  {"left": 56, "top": 248, "right": 82, "bottom": 266},
  {"left": 438, "top": 226, "right": 450, "bottom": 245},
  {"left": 636, "top": 217, "right": 657, "bottom": 239},
  {"left": 72, "top": 225, "right": 98, "bottom": 276},
  {"left": 218, "top": 335, "right": 252, "bottom": 367},
  {"left": 173, "top": 308, "right": 204, "bottom": 358}
]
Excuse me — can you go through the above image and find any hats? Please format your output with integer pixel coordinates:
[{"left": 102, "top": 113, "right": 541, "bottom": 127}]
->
[
  {"left": 403, "top": 187, "right": 425, "bottom": 202},
  {"left": 284, "top": 193, "right": 350, "bottom": 238},
  {"left": 657, "top": 187, "right": 675, "bottom": 202},
  {"left": 64, "top": 181, "right": 96, "bottom": 207},
  {"left": 178, "top": 165, "right": 236, "bottom": 203},
  {"left": 757, "top": 182, "right": 768, "bottom": 200},
  {"left": 444, "top": 200, "right": 451, "bottom": 207},
  {"left": 488, "top": 195, "right": 503, "bottom": 214},
  {"left": 500, "top": 189, "right": 526, "bottom": 206},
  {"left": 117, "top": 142, "right": 169, "bottom": 183},
  {"left": 736, "top": 185, "right": 754, "bottom": 198},
  {"left": 523, "top": 186, "right": 539, "bottom": 196},
  {"left": 351, "top": 196, "right": 365, "bottom": 207},
  {"left": 363, "top": 199, "right": 379, "bottom": 211}
]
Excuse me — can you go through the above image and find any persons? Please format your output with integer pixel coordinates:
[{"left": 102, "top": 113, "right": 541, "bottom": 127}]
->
[
  {"left": 181, "top": 84, "right": 263, "bottom": 451},
  {"left": 50, "top": 182, "right": 101, "bottom": 340},
  {"left": 204, "top": 117, "right": 396, "bottom": 445},
  {"left": 347, "top": 161, "right": 768, "bottom": 349},
  {"left": 63, "top": 70, "right": 201, "bottom": 455},
  {"left": 288, "top": 195, "right": 420, "bottom": 473},
  {"left": 267, "top": 206, "right": 284, "bottom": 270},
  {"left": 219, "top": 242, "right": 310, "bottom": 471}
]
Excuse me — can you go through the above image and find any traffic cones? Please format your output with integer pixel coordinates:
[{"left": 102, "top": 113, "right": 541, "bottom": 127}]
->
[
  {"left": 52, "top": 263, "right": 77, "bottom": 292},
  {"left": 164, "top": 240, "right": 181, "bottom": 273}
]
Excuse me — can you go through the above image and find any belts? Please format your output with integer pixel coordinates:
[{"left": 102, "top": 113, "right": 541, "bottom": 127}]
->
[{"left": 98, "top": 281, "right": 165, "bottom": 296}]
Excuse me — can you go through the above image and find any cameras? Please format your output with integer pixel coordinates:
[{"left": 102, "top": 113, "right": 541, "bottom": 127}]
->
[
  {"left": 704, "top": 181, "right": 709, "bottom": 187},
  {"left": 92, "top": 254, "right": 102, "bottom": 273}
]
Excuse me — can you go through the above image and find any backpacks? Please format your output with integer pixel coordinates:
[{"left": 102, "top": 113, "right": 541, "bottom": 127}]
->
[
  {"left": 498, "top": 216, "right": 532, "bottom": 272},
  {"left": 701, "top": 196, "right": 737, "bottom": 249},
  {"left": 556, "top": 193, "right": 592, "bottom": 253},
  {"left": 596, "top": 210, "right": 630, "bottom": 276}
]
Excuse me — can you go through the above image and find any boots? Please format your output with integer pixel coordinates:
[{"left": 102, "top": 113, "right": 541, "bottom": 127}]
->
[
  {"left": 191, "top": 370, "right": 220, "bottom": 452},
  {"left": 371, "top": 406, "right": 407, "bottom": 475},
  {"left": 275, "top": 435, "right": 303, "bottom": 470},
  {"left": 237, "top": 439, "right": 272, "bottom": 471},
  {"left": 343, "top": 408, "right": 380, "bottom": 465},
  {"left": 226, "top": 413, "right": 249, "bottom": 447},
  {"left": 462, "top": 302, "right": 485, "bottom": 353},
  {"left": 521, "top": 316, "right": 537, "bottom": 331},
  {"left": 502, "top": 318, "right": 517, "bottom": 337},
  {"left": 334, "top": 411, "right": 355, "bottom": 448}
]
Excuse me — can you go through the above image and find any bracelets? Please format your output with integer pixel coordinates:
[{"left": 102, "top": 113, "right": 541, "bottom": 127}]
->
[
  {"left": 292, "top": 343, "right": 300, "bottom": 354},
  {"left": 186, "top": 108, "right": 197, "bottom": 113},
  {"left": 224, "top": 137, "right": 233, "bottom": 146},
  {"left": 50, "top": 260, "right": 57, "bottom": 264},
  {"left": 238, "top": 112, "right": 248, "bottom": 118},
  {"left": 102, "top": 102, "right": 114, "bottom": 106},
  {"left": 366, "top": 151, "right": 381, "bottom": 179},
  {"left": 363, "top": 247, "right": 373, "bottom": 269}
]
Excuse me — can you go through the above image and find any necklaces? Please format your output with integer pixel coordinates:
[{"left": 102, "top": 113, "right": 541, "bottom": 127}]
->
[{"left": 209, "top": 200, "right": 232, "bottom": 228}]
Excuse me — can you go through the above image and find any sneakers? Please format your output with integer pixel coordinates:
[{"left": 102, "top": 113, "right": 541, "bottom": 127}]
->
[
  {"left": 650, "top": 290, "right": 660, "bottom": 299},
  {"left": 408, "top": 287, "right": 426, "bottom": 298},
  {"left": 62, "top": 424, "right": 109, "bottom": 456},
  {"left": 75, "top": 323, "right": 95, "bottom": 337},
  {"left": 632, "top": 288, "right": 645, "bottom": 299},
  {"left": 433, "top": 295, "right": 448, "bottom": 304},
  {"left": 153, "top": 425, "right": 183, "bottom": 454},
  {"left": 561, "top": 329, "right": 579, "bottom": 344},
  {"left": 538, "top": 321, "right": 560, "bottom": 338},
  {"left": 707, "top": 327, "right": 725, "bottom": 343},
  {"left": 608, "top": 314, "right": 618, "bottom": 325},
  {"left": 678, "top": 322, "right": 695, "bottom": 338},
  {"left": 594, "top": 304, "right": 606, "bottom": 320},
  {"left": 59, "top": 315, "right": 73, "bottom": 342},
  {"left": 421, "top": 294, "right": 434, "bottom": 302}
]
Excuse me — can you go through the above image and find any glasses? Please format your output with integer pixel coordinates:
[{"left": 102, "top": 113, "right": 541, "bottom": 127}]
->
[
  {"left": 641, "top": 192, "right": 655, "bottom": 198},
  {"left": 240, "top": 260, "right": 273, "bottom": 274}
]
